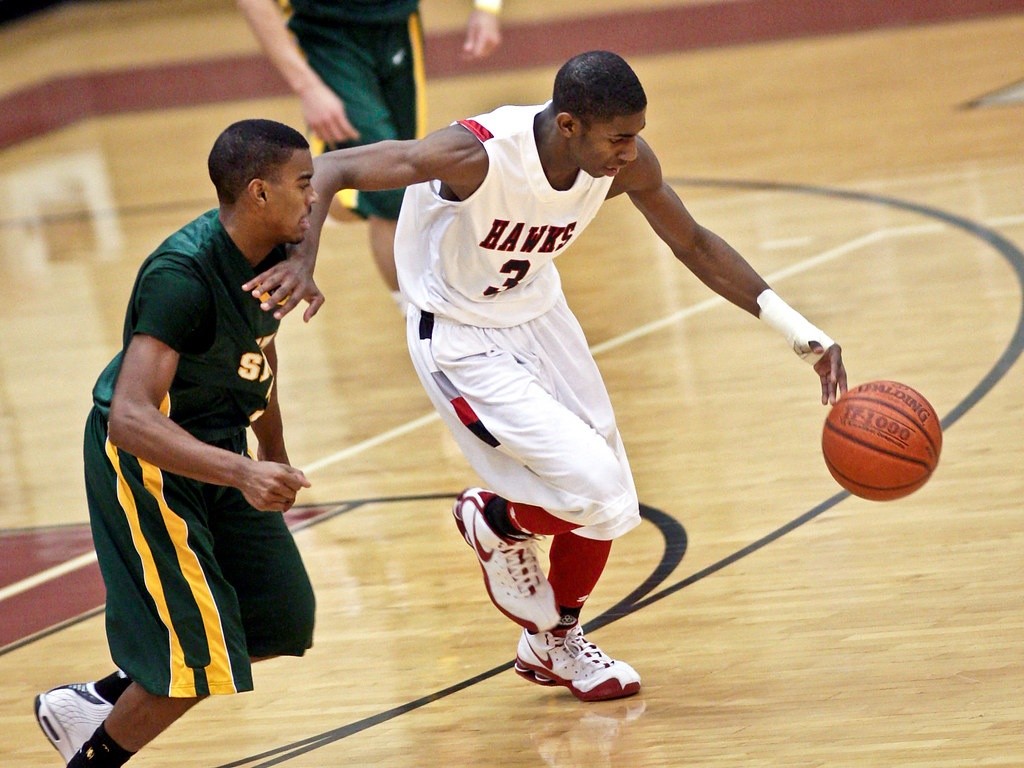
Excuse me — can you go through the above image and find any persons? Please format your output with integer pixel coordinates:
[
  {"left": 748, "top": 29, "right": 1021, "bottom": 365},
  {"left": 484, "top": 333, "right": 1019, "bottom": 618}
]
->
[
  {"left": 241, "top": 50, "right": 847, "bottom": 702},
  {"left": 237, "top": 0, "right": 505, "bottom": 321},
  {"left": 33, "top": 120, "right": 315, "bottom": 768}
]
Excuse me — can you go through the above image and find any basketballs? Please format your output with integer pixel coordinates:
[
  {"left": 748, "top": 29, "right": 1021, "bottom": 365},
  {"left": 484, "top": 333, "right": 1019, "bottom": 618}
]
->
[{"left": 819, "top": 377, "right": 944, "bottom": 503}]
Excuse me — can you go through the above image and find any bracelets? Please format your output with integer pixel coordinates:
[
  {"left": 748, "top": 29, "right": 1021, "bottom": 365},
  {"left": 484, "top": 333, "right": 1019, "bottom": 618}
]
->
[{"left": 471, "top": 0, "right": 504, "bottom": 16}]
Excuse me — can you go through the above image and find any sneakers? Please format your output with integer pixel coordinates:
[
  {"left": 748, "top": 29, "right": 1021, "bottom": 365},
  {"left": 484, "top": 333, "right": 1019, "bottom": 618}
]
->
[
  {"left": 452, "top": 486, "right": 560, "bottom": 632},
  {"left": 34, "top": 682, "right": 115, "bottom": 766},
  {"left": 515, "top": 621, "right": 640, "bottom": 702}
]
